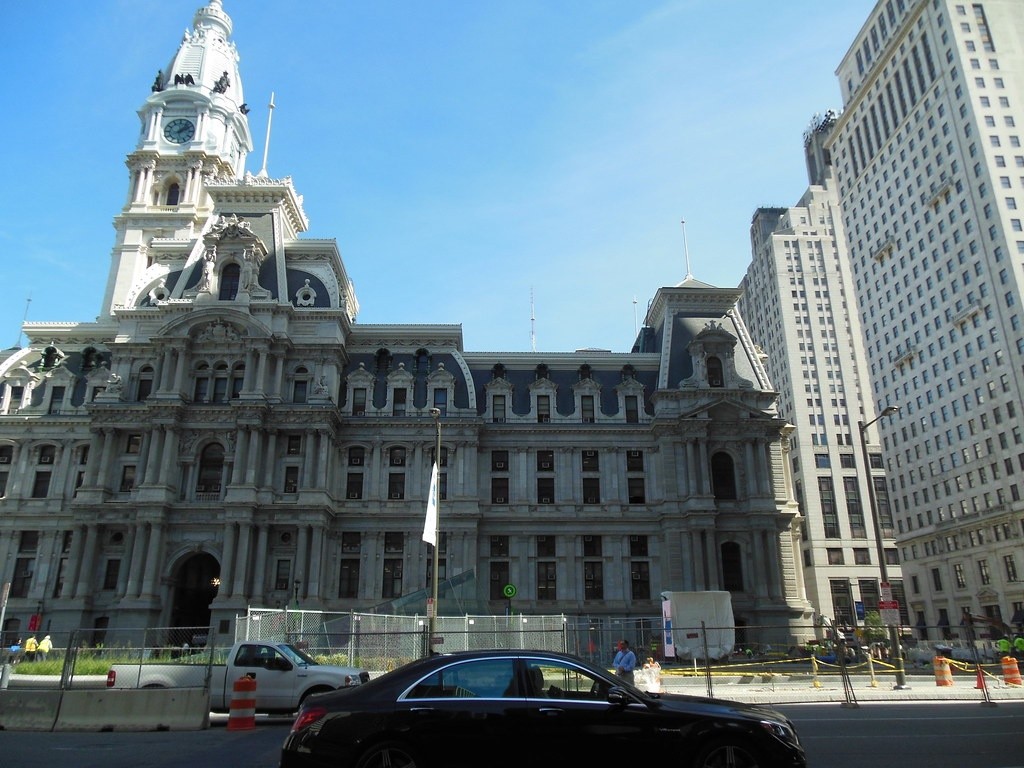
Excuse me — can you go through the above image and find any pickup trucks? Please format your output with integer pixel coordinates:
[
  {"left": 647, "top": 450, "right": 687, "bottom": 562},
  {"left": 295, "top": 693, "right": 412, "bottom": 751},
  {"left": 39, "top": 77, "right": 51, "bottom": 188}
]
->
[{"left": 105, "top": 639, "right": 371, "bottom": 718}]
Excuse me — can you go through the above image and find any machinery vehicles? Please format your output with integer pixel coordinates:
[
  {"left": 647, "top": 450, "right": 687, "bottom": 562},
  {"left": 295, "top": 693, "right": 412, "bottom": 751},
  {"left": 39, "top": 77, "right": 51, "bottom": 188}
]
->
[{"left": 958, "top": 613, "right": 1024, "bottom": 662}]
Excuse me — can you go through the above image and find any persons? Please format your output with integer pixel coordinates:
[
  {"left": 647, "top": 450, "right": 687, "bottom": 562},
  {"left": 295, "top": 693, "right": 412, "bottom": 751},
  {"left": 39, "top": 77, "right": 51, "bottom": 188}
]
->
[
  {"left": 314, "top": 374, "right": 329, "bottom": 394},
  {"left": 199, "top": 322, "right": 240, "bottom": 340},
  {"left": 1013, "top": 634, "right": 1024, "bottom": 657},
  {"left": 196, "top": 250, "right": 215, "bottom": 291},
  {"left": 241, "top": 248, "right": 253, "bottom": 290},
  {"left": 213, "top": 70, "right": 231, "bottom": 94},
  {"left": 25, "top": 634, "right": 39, "bottom": 662},
  {"left": 530, "top": 664, "right": 565, "bottom": 699},
  {"left": 997, "top": 635, "right": 1012, "bottom": 660},
  {"left": 613, "top": 640, "right": 636, "bottom": 686},
  {"left": 106, "top": 373, "right": 123, "bottom": 392},
  {"left": 36, "top": 635, "right": 52, "bottom": 663},
  {"left": 152, "top": 68, "right": 165, "bottom": 92},
  {"left": 9, "top": 637, "right": 22, "bottom": 663}
]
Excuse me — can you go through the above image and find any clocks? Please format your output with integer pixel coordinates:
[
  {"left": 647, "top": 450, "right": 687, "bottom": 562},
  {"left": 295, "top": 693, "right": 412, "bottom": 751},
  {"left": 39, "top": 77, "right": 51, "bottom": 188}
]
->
[{"left": 164, "top": 119, "right": 195, "bottom": 144}]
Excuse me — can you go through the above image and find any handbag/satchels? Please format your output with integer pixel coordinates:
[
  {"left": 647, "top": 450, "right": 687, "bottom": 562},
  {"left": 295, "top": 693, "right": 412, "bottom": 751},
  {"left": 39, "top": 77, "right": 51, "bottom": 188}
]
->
[{"left": 633, "top": 662, "right": 661, "bottom": 693}]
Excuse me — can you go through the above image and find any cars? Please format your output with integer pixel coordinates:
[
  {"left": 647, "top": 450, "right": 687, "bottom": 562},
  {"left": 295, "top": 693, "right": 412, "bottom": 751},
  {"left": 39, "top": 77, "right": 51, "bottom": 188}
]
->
[{"left": 274, "top": 647, "right": 808, "bottom": 768}]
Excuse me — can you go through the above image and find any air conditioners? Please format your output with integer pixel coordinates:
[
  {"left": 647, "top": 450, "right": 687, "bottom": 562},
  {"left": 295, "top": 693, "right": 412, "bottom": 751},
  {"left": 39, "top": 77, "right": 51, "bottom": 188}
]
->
[
  {"left": 491, "top": 573, "right": 499, "bottom": 580},
  {"left": 586, "top": 574, "right": 594, "bottom": 580},
  {"left": 496, "top": 462, "right": 503, "bottom": 468},
  {"left": 427, "top": 571, "right": 432, "bottom": 577},
  {"left": 538, "top": 535, "right": 545, "bottom": 541},
  {"left": 542, "top": 463, "right": 549, "bottom": 468},
  {"left": 583, "top": 417, "right": 590, "bottom": 423},
  {"left": 548, "top": 574, "right": 555, "bottom": 580},
  {"left": 393, "top": 572, "right": 401, "bottom": 578},
  {"left": 543, "top": 498, "right": 549, "bottom": 503},
  {"left": 631, "top": 535, "right": 638, "bottom": 542},
  {"left": 589, "top": 497, "right": 596, "bottom": 503},
  {"left": 22, "top": 571, "right": 31, "bottom": 577},
  {"left": 585, "top": 535, "right": 592, "bottom": 541},
  {"left": 212, "top": 485, "right": 220, "bottom": 491},
  {"left": 357, "top": 411, "right": 364, "bottom": 417},
  {"left": 498, "top": 417, "right": 505, "bottom": 423},
  {"left": 352, "top": 458, "right": 360, "bottom": 464},
  {"left": 41, "top": 457, "right": 50, "bottom": 462},
  {"left": 631, "top": 451, "right": 638, "bottom": 456},
  {"left": 633, "top": 573, "right": 640, "bottom": 580},
  {"left": 84, "top": 456, "right": 87, "bottom": 462},
  {"left": 496, "top": 496, "right": 504, "bottom": 503},
  {"left": 350, "top": 493, "right": 357, "bottom": 498},
  {"left": 51, "top": 409, "right": 59, "bottom": 414},
  {"left": 587, "top": 451, "right": 595, "bottom": 456},
  {"left": 10, "top": 409, "right": 18, "bottom": 414},
  {"left": 440, "top": 459, "right": 442, "bottom": 464},
  {"left": 490, "top": 535, "right": 498, "bottom": 541},
  {"left": 394, "top": 459, "right": 401, "bottom": 464},
  {"left": 543, "top": 418, "right": 549, "bottom": 423},
  {"left": 392, "top": 493, "right": 400, "bottom": 498},
  {"left": 0, "top": 457, "right": 8, "bottom": 462},
  {"left": 197, "top": 485, "right": 204, "bottom": 491}
]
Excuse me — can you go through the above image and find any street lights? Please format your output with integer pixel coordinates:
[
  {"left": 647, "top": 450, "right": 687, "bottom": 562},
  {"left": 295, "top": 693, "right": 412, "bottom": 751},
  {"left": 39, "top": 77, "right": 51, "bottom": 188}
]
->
[
  {"left": 858, "top": 406, "right": 910, "bottom": 689},
  {"left": 428, "top": 405, "right": 447, "bottom": 654}
]
